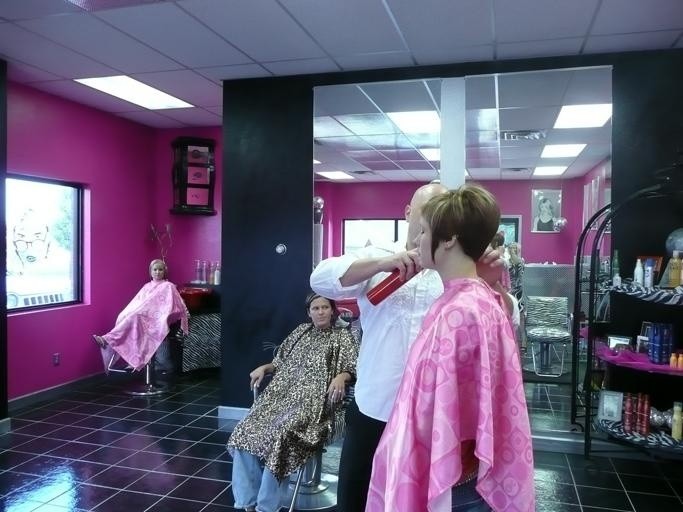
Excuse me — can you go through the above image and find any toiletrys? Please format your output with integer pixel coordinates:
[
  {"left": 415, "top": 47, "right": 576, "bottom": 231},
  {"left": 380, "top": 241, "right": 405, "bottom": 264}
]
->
[
  {"left": 678, "top": 354, "right": 683, "bottom": 370},
  {"left": 612, "top": 273, "right": 622, "bottom": 288},
  {"left": 670, "top": 353, "right": 677, "bottom": 368}
]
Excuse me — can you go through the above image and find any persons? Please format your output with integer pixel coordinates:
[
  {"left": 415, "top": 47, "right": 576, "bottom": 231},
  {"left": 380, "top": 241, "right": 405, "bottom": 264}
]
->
[
  {"left": 93, "top": 258, "right": 191, "bottom": 371},
  {"left": 361, "top": 186, "right": 535, "bottom": 512},
  {"left": 309, "top": 182, "right": 519, "bottom": 512},
  {"left": 534, "top": 198, "right": 558, "bottom": 231},
  {"left": 491, "top": 234, "right": 512, "bottom": 294},
  {"left": 227, "top": 292, "right": 358, "bottom": 512},
  {"left": 507, "top": 242, "right": 529, "bottom": 351}
]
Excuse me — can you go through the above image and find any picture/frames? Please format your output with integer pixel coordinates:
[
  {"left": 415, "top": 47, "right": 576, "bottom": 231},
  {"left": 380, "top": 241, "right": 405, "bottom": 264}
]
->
[
  {"left": 597, "top": 389, "right": 624, "bottom": 423},
  {"left": 530, "top": 188, "right": 563, "bottom": 234},
  {"left": 607, "top": 320, "right": 654, "bottom": 356},
  {"left": 636, "top": 255, "right": 663, "bottom": 285},
  {"left": 498, "top": 214, "right": 523, "bottom": 259}
]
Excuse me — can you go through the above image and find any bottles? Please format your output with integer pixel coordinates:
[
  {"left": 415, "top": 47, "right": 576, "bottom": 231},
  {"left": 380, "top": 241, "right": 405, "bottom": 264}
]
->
[
  {"left": 644, "top": 258, "right": 654, "bottom": 288},
  {"left": 669, "top": 353, "right": 677, "bottom": 368},
  {"left": 669, "top": 250, "right": 681, "bottom": 288},
  {"left": 634, "top": 258, "right": 643, "bottom": 286},
  {"left": 648, "top": 323, "right": 674, "bottom": 364},
  {"left": 623, "top": 392, "right": 651, "bottom": 435},
  {"left": 611, "top": 248, "right": 620, "bottom": 280},
  {"left": 671, "top": 401, "right": 682, "bottom": 442},
  {"left": 208, "top": 263, "right": 220, "bottom": 285},
  {"left": 677, "top": 353, "right": 682, "bottom": 368}
]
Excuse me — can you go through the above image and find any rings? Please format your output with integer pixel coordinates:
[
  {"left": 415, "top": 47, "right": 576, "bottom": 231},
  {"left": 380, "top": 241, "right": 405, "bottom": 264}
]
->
[{"left": 338, "top": 390, "right": 341, "bottom": 392}]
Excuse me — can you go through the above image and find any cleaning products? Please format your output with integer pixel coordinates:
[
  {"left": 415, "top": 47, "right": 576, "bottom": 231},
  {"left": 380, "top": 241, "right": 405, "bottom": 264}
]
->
[
  {"left": 612, "top": 250, "right": 622, "bottom": 286},
  {"left": 668, "top": 249, "right": 683, "bottom": 287}
]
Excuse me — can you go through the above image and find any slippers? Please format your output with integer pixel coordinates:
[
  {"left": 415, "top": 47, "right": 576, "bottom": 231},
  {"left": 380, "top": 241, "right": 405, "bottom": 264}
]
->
[
  {"left": 125, "top": 367, "right": 134, "bottom": 371},
  {"left": 91, "top": 335, "right": 108, "bottom": 349}
]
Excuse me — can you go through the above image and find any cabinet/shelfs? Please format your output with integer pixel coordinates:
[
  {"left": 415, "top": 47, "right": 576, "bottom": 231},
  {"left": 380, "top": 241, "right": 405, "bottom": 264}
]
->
[{"left": 571, "top": 180, "right": 683, "bottom": 464}]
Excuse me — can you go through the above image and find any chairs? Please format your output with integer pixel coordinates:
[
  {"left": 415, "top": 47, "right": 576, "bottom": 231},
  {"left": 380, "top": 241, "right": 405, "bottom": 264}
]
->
[
  {"left": 108, "top": 320, "right": 184, "bottom": 395},
  {"left": 252, "top": 369, "right": 339, "bottom": 512},
  {"left": 525, "top": 296, "right": 576, "bottom": 377}
]
